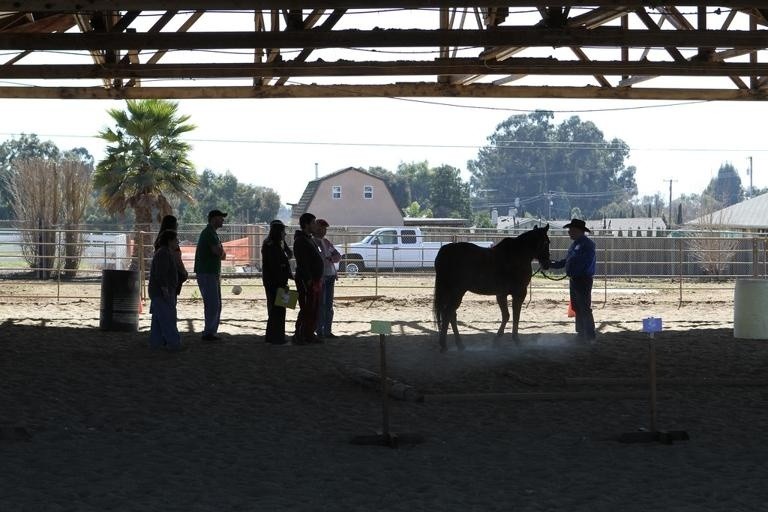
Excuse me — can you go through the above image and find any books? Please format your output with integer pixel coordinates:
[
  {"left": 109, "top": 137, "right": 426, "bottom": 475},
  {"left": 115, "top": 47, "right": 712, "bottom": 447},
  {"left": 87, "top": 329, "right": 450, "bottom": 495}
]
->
[{"left": 273, "top": 286, "right": 299, "bottom": 309}]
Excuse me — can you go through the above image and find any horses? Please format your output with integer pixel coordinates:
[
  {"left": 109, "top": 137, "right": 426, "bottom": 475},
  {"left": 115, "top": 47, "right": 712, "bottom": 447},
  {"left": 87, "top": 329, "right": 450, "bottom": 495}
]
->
[{"left": 431, "top": 224, "right": 552, "bottom": 355}]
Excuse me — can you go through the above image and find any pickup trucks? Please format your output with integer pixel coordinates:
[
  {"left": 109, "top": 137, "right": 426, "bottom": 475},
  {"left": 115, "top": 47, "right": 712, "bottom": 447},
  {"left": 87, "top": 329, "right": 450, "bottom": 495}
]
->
[{"left": 329, "top": 227, "right": 494, "bottom": 275}]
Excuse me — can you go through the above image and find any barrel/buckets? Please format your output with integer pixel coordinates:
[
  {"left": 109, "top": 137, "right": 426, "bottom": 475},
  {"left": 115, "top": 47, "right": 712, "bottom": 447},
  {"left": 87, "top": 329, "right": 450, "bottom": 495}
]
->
[
  {"left": 734, "top": 278, "right": 768, "bottom": 340},
  {"left": 100, "top": 270, "right": 142, "bottom": 333}
]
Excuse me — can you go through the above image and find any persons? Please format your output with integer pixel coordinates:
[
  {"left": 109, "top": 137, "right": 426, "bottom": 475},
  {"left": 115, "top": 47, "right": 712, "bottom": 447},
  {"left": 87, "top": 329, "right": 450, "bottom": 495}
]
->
[
  {"left": 146, "top": 229, "right": 188, "bottom": 352},
  {"left": 148, "top": 214, "right": 187, "bottom": 346},
  {"left": 261, "top": 219, "right": 295, "bottom": 280},
  {"left": 193, "top": 210, "right": 227, "bottom": 343},
  {"left": 547, "top": 218, "right": 596, "bottom": 345},
  {"left": 312, "top": 219, "right": 342, "bottom": 339},
  {"left": 261, "top": 223, "right": 289, "bottom": 345},
  {"left": 293, "top": 212, "right": 326, "bottom": 344}
]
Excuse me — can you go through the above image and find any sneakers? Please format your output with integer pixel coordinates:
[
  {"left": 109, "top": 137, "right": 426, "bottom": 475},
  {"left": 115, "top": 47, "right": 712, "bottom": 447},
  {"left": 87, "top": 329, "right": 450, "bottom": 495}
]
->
[
  {"left": 202, "top": 330, "right": 218, "bottom": 339},
  {"left": 263, "top": 331, "right": 337, "bottom": 344},
  {"left": 576, "top": 333, "right": 595, "bottom": 340},
  {"left": 148, "top": 342, "right": 186, "bottom": 353}
]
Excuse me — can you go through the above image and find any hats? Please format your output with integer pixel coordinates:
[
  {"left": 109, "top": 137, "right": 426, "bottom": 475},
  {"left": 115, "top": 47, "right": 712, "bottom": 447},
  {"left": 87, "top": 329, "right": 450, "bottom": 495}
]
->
[
  {"left": 316, "top": 219, "right": 329, "bottom": 227},
  {"left": 208, "top": 210, "right": 227, "bottom": 217},
  {"left": 270, "top": 219, "right": 288, "bottom": 227},
  {"left": 563, "top": 218, "right": 590, "bottom": 232}
]
